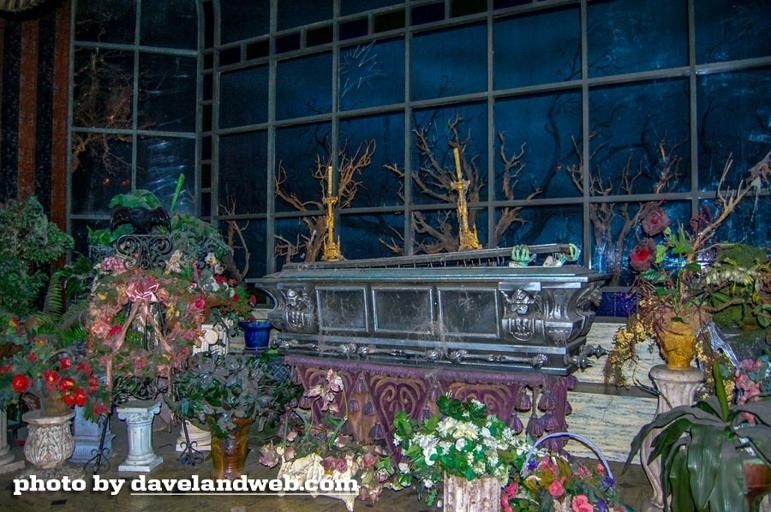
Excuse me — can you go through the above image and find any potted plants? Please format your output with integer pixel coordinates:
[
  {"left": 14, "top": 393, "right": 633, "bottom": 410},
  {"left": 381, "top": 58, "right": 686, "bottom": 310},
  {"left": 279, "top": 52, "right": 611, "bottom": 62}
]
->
[
  {"left": 620, "top": 358, "right": 771, "bottom": 512},
  {"left": 171, "top": 354, "right": 304, "bottom": 480}
]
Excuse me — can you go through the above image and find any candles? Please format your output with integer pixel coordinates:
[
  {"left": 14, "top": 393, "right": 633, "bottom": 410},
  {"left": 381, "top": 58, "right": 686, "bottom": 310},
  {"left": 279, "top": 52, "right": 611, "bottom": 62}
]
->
[
  {"left": 453, "top": 147, "right": 463, "bottom": 179},
  {"left": 327, "top": 166, "right": 333, "bottom": 196}
]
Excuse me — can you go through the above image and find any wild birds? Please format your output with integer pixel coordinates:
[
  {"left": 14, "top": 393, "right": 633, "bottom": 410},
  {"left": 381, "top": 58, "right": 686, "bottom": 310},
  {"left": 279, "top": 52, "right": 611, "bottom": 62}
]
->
[{"left": 108, "top": 205, "right": 172, "bottom": 235}]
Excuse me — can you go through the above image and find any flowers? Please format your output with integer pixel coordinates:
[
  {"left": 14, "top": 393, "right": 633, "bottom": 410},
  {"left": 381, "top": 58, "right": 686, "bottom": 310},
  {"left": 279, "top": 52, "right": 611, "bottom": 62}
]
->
[
  {"left": 393, "top": 391, "right": 569, "bottom": 488},
  {"left": 604, "top": 206, "right": 771, "bottom": 327},
  {"left": 500, "top": 454, "right": 626, "bottom": 512},
  {"left": 260, "top": 366, "right": 391, "bottom": 502},
  {"left": 166, "top": 249, "right": 257, "bottom": 321},
  {"left": 87, "top": 268, "right": 205, "bottom": 377},
  {"left": 0, "top": 316, "right": 113, "bottom": 416}
]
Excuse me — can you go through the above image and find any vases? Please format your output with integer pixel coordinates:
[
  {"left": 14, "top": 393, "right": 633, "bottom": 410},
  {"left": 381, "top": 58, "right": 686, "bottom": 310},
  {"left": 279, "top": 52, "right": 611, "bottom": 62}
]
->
[
  {"left": 22, "top": 409, "right": 76, "bottom": 469},
  {"left": 238, "top": 320, "right": 274, "bottom": 351},
  {"left": 277, "top": 452, "right": 360, "bottom": 512},
  {"left": 443, "top": 470, "right": 502, "bottom": 512},
  {"left": 651, "top": 311, "right": 711, "bottom": 369}
]
